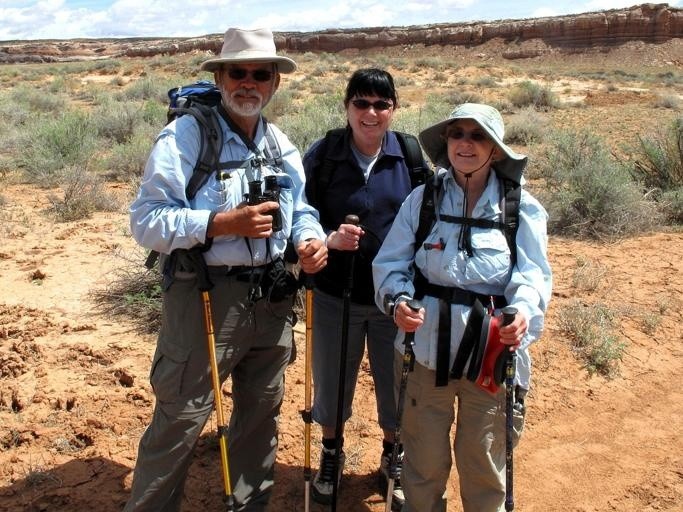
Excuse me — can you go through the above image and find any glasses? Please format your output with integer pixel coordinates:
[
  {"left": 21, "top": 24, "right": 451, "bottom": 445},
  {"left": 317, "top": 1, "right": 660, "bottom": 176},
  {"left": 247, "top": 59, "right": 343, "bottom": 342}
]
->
[
  {"left": 350, "top": 99, "right": 391, "bottom": 109},
  {"left": 228, "top": 68, "right": 274, "bottom": 80},
  {"left": 446, "top": 129, "right": 484, "bottom": 140}
]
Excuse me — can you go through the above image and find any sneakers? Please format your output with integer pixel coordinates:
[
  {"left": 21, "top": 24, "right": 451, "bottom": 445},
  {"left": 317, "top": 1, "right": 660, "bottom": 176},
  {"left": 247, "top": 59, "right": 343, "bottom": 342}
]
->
[
  {"left": 310, "top": 447, "right": 344, "bottom": 505},
  {"left": 378, "top": 450, "right": 405, "bottom": 510}
]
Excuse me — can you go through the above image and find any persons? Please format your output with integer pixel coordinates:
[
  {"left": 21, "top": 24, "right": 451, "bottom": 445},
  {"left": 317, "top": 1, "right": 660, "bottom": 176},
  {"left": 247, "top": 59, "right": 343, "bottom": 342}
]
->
[
  {"left": 298, "top": 69, "right": 433, "bottom": 506},
  {"left": 120, "top": 25, "right": 332, "bottom": 511},
  {"left": 371, "top": 100, "right": 557, "bottom": 512}
]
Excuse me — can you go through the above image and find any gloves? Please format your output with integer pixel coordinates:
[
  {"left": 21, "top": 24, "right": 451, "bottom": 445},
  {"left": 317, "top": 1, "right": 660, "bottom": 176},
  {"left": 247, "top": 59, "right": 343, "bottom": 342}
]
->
[{"left": 165, "top": 80, "right": 222, "bottom": 201}]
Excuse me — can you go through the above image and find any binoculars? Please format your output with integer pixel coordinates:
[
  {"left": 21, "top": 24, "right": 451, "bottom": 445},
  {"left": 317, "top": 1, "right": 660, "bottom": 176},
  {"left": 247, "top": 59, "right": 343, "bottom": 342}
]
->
[{"left": 244, "top": 176, "right": 283, "bottom": 232}]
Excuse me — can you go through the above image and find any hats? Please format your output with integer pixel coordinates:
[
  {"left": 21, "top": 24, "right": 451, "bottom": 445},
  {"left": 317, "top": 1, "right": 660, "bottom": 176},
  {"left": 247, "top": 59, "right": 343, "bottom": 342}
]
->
[
  {"left": 200, "top": 27, "right": 296, "bottom": 73},
  {"left": 419, "top": 102, "right": 527, "bottom": 186}
]
